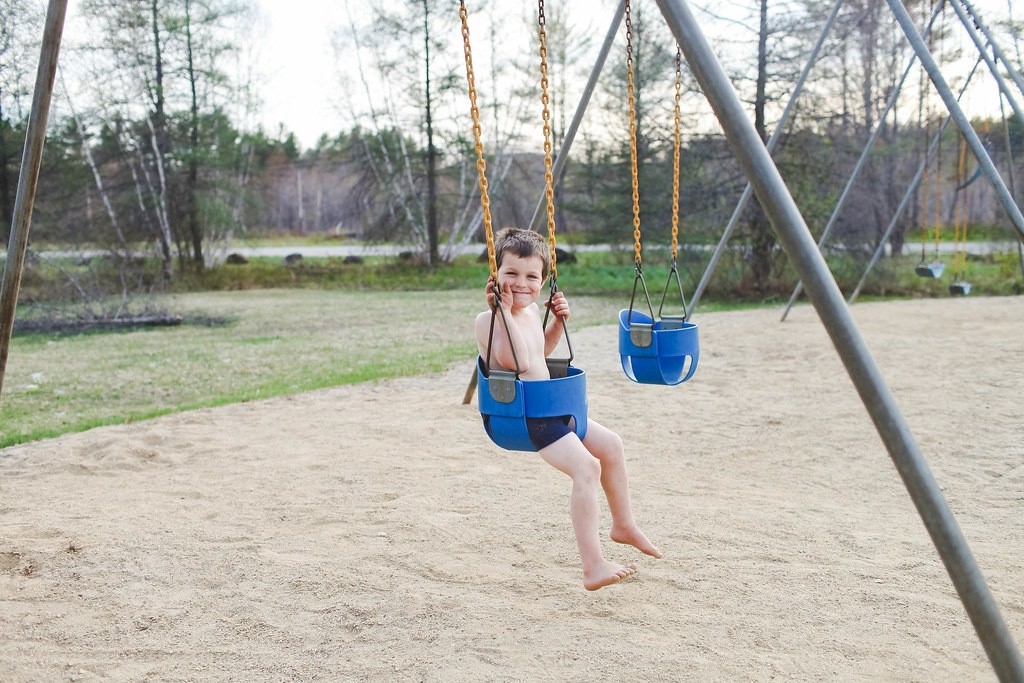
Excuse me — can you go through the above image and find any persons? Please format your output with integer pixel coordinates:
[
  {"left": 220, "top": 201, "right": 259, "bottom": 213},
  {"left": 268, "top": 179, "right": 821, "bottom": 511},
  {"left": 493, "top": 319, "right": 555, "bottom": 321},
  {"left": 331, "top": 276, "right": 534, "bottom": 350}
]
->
[{"left": 476, "top": 229, "right": 662, "bottom": 591}]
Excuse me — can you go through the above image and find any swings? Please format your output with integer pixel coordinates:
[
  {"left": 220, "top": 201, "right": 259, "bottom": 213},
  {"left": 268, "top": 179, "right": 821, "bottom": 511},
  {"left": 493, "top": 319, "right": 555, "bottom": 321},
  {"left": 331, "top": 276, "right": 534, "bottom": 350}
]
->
[
  {"left": 452, "top": 1, "right": 587, "bottom": 450},
  {"left": 618, "top": 0, "right": 699, "bottom": 386},
  {"left": 912, "top": 1, "right": 976, "bottom": 300}
]
